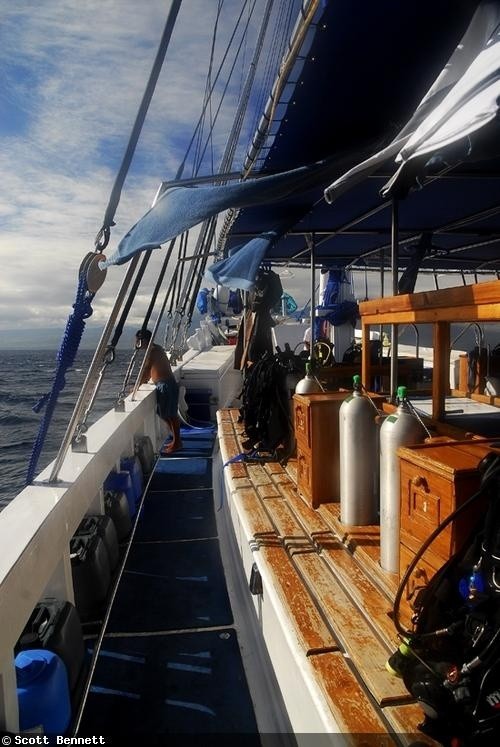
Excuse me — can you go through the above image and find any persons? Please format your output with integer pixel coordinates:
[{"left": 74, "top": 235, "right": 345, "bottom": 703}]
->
[{"left": 136, "top": 329, "right": 183, "bottom": 454}]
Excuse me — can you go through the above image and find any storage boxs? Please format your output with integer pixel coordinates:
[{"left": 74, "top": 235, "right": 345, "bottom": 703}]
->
[
  {"left": 187, "top": 403, "right": 212, "bottom": 423},
  {"left": 185, "top": 387, "right": 213, "bottom": 403}
]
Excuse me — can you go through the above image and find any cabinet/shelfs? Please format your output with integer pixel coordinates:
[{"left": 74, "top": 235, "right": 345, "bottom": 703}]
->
[
  {"left": 397, "top": 439, "right": 500, "bottom": 635},
  {"left": 292, "top": 391, "right": 387, "bottom": 509}
]
